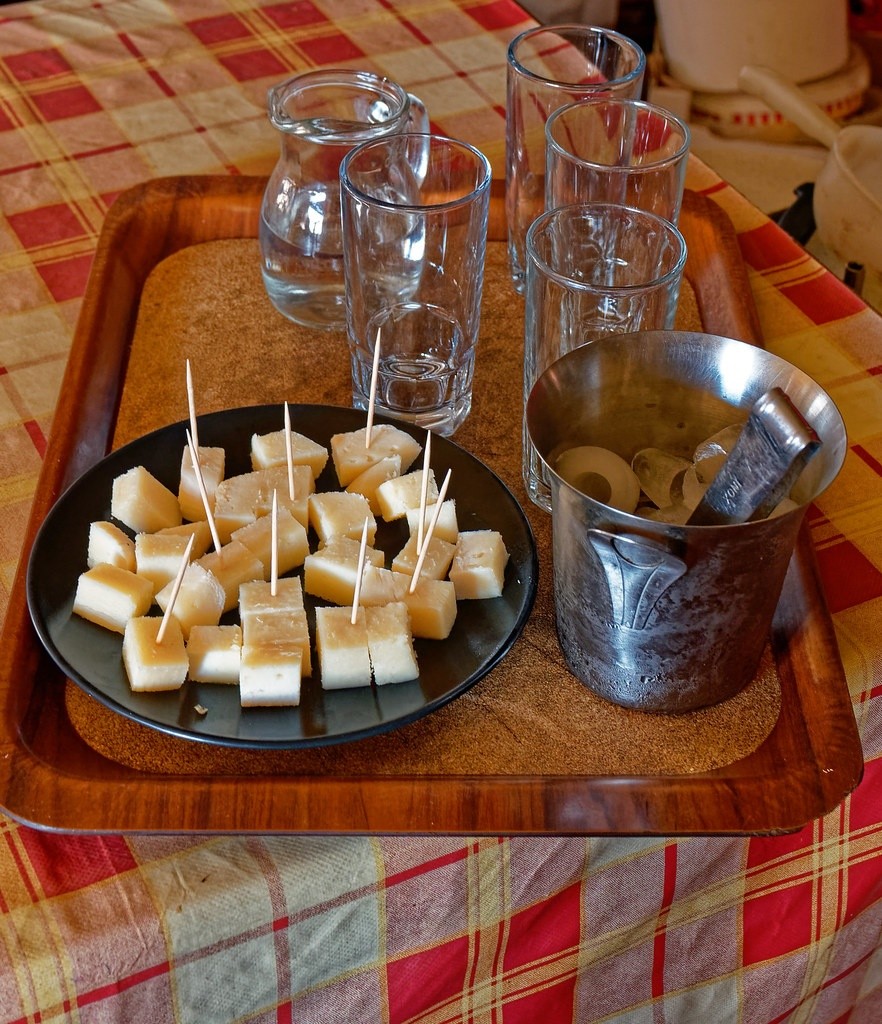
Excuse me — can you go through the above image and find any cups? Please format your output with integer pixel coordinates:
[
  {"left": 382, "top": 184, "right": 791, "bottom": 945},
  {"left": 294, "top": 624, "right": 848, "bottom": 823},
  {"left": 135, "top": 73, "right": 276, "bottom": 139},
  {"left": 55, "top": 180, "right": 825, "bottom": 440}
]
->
[
  {"left": 524, "top": 328, "right": 850, "bottom": 714},
  {"left": 544, "top": 95, "right": 691, "bottom": 228},
  {"left": 337, "top": 130, "right": 494, "bottom": 438},
  {"left": 522, "top": 201, "right": 688, "bottom": 515},
  {"left": 503, "top": 22, "right": 647, "bottom": 297}
]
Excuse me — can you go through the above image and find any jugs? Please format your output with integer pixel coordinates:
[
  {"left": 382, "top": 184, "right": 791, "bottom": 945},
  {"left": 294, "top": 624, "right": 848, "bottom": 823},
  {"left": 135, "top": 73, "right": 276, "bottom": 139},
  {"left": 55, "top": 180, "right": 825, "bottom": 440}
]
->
[{"left": 257, "top": 68, "right": 431, "bottom": 334}]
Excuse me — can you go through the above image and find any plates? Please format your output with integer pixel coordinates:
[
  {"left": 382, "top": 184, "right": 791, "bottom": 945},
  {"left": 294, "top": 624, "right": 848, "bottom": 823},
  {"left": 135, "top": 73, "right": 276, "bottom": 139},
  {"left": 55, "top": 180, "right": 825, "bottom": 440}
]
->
[{"left": 26, "top": 404, "right": 540, "bottom": 751}]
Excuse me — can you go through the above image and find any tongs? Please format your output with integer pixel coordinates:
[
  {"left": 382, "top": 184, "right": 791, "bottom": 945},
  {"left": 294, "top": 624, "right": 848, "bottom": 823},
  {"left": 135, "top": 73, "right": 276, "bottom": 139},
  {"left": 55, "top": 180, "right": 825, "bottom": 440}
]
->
[{"left": 687, "top": 387, "right": 821, "bottom": 525}]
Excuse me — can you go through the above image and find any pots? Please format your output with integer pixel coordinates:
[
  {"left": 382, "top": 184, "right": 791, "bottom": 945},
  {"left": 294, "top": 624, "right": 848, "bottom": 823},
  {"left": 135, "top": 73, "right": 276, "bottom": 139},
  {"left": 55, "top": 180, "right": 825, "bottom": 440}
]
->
[{"left": 738, "top": 62, "right": 882, "bottom": 278}]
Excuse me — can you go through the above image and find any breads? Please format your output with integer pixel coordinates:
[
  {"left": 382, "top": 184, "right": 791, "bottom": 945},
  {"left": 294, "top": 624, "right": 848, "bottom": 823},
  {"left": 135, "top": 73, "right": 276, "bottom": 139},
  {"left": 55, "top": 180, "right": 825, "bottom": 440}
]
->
[{"left": 74, "top": 426, "right": 508, "bottom": 705}]
